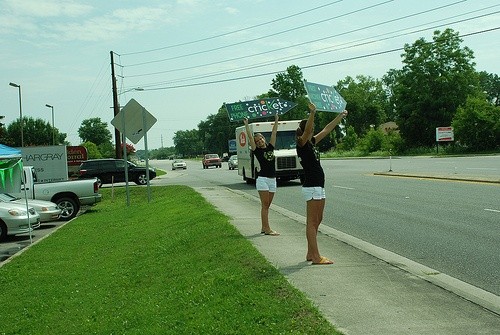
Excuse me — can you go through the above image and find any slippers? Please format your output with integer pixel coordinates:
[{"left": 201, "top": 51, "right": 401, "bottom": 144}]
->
[
  {"left": 307, "top": 259, "right": 312, "bottom": 261},
  {"left": 312, "top": 257, "right": 333, "bottom": 264},
  {"left": 265, "top": 230, "right": 280, "bottom": 235},
  {"left": 261, "top": 231, "right": 265, "bottom": 234}
]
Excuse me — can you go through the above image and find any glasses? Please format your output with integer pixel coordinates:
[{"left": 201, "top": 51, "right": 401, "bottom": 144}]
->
[{"left": 255, "top": 137, "right": 263, "bottom": 142}]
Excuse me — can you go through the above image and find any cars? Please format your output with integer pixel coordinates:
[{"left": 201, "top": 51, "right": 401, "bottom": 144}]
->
[
  {"left": 172, "top": 159, "right": 187, "bottom": 170},
  {"left": 0, "top": 192, "right": 62, "bottom": 222},
  {"left": 0, "top": 194, "right": 41, "bottom": 242},
  {"left": 227, "top": 155, "right": 238, "bottom": 170}
]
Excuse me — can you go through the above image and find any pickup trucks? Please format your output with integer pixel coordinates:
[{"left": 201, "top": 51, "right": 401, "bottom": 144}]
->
[{"left": 6, "top": 165, "right": 102, "bottom": 221}]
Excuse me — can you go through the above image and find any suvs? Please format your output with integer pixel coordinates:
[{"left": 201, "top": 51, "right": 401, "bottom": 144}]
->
[
  {"left": 77, "top": 158, "right": 157, "bottom": 185},
  {"left": 202, "top": 154, "right": 222, "bottom": 169}
]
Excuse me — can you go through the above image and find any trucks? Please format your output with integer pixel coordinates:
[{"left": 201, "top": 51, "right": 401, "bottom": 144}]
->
[{"left": 235, "top": 120, "right": 322, "bottom": 182}]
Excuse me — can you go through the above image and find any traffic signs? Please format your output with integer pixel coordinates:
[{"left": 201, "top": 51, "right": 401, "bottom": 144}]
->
[
  {"left": 303, "top": 80, "right": 348, "bottom": 113},
  {"left": 225, "top": 97, "right": 299, "bottom": 123}
]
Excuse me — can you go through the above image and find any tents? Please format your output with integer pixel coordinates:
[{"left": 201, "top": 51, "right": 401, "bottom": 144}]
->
[{"left": 0, "top": 143, "right": 33, "bottom": 245}]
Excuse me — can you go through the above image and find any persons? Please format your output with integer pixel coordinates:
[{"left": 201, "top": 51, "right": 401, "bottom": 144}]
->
[
  {"left": 243, "top": 113, "right": 279, "bottom": 236},
  {"left": 296, "top": 104, "right": 348, "bottom": 265}
]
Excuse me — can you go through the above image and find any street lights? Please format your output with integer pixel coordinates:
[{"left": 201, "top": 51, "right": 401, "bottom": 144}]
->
[
  {"left": 45, "top": 104, "right": 55, "bottom": 146},
  {"left": 112, "top": 87, "right": 145, "bottom": 159},
  {"left": 9, "top": 81, "right": 25, "bottom": 167}
]
